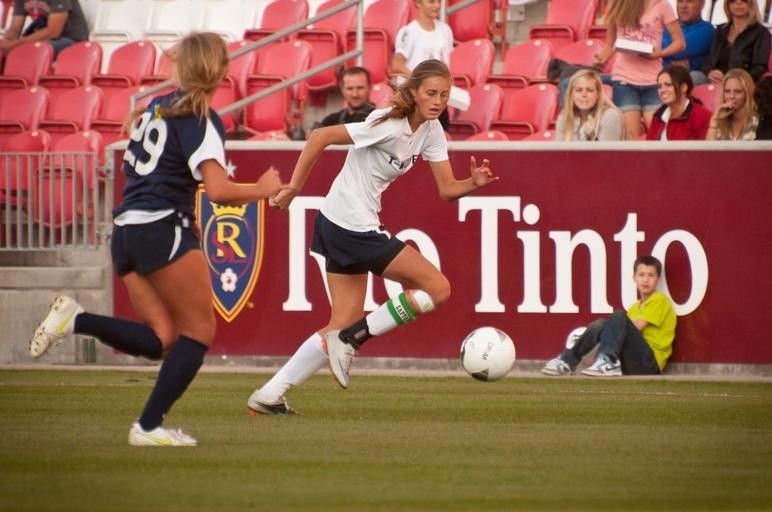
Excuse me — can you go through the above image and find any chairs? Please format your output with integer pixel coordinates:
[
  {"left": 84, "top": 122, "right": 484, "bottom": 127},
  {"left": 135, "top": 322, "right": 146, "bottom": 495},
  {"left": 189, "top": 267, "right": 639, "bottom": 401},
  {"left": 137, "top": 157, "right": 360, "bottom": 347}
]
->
[{"left": 0, "top": 0, "right": 772, "bottom": 247}]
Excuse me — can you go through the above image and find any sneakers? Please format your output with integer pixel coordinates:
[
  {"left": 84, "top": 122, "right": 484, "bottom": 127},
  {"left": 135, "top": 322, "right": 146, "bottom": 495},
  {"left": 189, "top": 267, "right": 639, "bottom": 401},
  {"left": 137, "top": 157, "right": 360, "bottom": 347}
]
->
[
  {"left": 581, "top": 354, "right": 624, "bottom": 377},
  {"left": 321, "top": 329, "right": 358, "bottom": 390},
  {"left": 28, "top": 295, "right": 84, "bottom": 358},
  {"left": 128, "top": 421, "right": 198, "bottom": 447},
  {"left": 248, "top": 389, "right": 300, "bottom": 417},
  {"left": 541, "top": 358, "right": 577, "bottom": 377}
]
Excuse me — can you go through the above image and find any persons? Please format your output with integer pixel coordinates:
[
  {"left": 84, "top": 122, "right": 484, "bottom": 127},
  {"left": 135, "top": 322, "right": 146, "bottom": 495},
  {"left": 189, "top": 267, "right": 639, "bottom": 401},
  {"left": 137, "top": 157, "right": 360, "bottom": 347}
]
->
[
  {"left": 391, "top": 0, "right": 454, "bottom": 132},
  {"left": 554, "top": 0, "right": 772, "bottom": 140},
  {"left": 248, "top": 60, "right": 500, "bottom": 412},
  {"left": 542, "top": 256, "right": 676, "bottom": 378},
  {"left": 306, "top": 68, "right": 376, "bottom": 141},
  {"left": 30, "top": 33, "right": 283, "bottom": 447},
  {"left": 0, "top": 0, "right": 90, "bottom": 61}
]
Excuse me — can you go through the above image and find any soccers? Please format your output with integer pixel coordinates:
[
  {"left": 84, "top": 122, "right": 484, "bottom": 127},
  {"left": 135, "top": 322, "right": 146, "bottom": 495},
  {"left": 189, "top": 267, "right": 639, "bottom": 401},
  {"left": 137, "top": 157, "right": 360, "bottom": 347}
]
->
[{"left": 460, "top": 328, "right": 516, "bottom": 381}]
